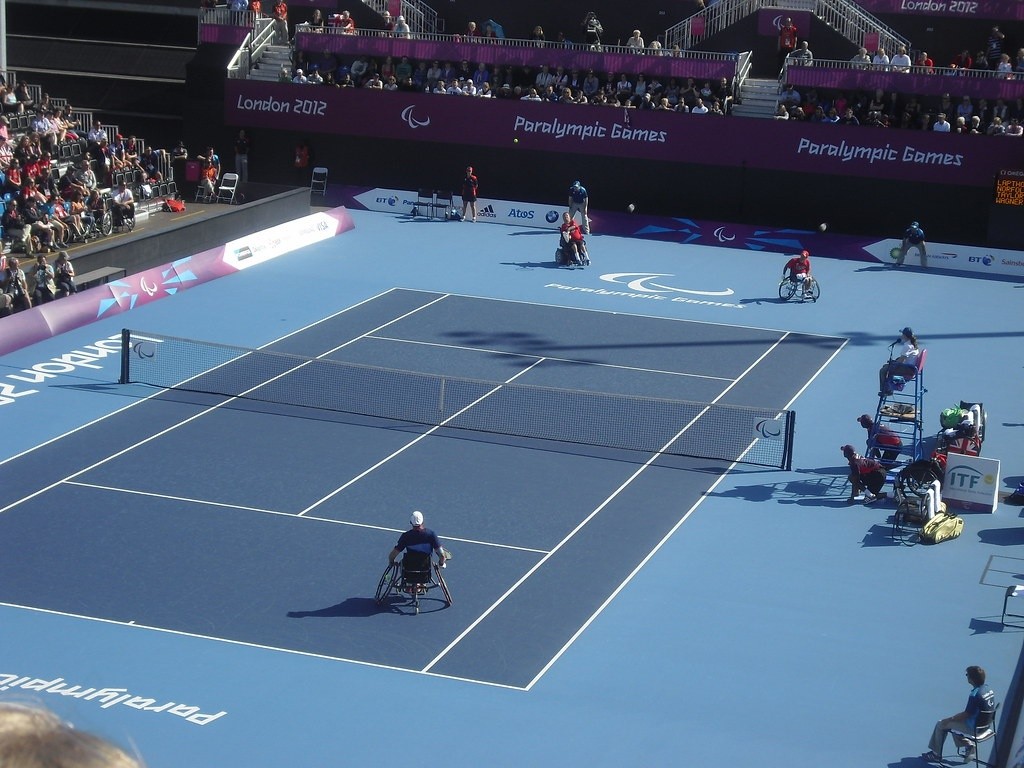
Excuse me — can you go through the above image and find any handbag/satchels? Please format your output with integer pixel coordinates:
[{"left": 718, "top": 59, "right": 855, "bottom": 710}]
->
[
  {"left": 940, "top": 402, "right": 969, "bottom": 430},
  {"left": 918, "top": 511, "right": 964, "bottom": 544}
]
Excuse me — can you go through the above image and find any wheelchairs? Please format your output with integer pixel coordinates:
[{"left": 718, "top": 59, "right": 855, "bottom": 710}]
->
[
  {"left": 555, "top": 226, "right": 587, "bottom": 266},
  {"left": 779, "top": 275, "right": 820, "bottom": 303},
  {"left": 892, "top": 400, "right": 987, "bottom": 547},
  {"left": 62, "top": 197, "right": 136, "bottom": 244},
  {"left": 375, "top": 553, "right": 453, "bottom": 616}
]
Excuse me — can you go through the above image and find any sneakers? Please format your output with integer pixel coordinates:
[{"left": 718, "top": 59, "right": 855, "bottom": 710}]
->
[
  {"left": 963, "top": 744, "right": 975, "bottom": 764},
  {"left": 921, "top": 752, "right": 941, "bottom": 762}
]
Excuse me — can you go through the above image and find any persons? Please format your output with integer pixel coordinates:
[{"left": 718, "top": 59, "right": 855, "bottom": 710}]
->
[
  {"left": 896, "top": 222, "right": 928, "bottom": 272},
  {"left": 857, "top": 414, "right": 912, "bottom": 468},
  {"left": 569, "top": 181, "right": 590, "bottom": 234},
  {"left": 0, "top": 700, "right": 141, "bottom": 768},
  {"left": 0, "top": 73, "right": 220, "bottom": 319},
  {"left": 840, "top": 445, "right": 900, "bottom": 504},
  {"left": 777, "top": 17, "right": 813, "bottom": 72},
  {"left": 279, "top": 11, "right": 731, "bottom": 114},
  {"left": 459, "top": 166, "right": 478, "bottom": 223},
  {"left": 878, "top": 327, "right": 920, "bottom": 396},
  {"left": 226, "top": 0, "right": 289, "bottom": 45},
  {"left": 922, "top": 666, "right": 994, "bottom": 763},
  {"left": 562, "top": 212, "right": 591, "bottom": 266},
  {"left": 234, "top": 130, "right": 250, "bottom": 184},
  {"left": 389, "top": 510, "right": 446, "bottom": 593},
  {"left": 850, "top": 26, "right": 1024, "bottom": 80},
  {"left": 783, "top": 251, "right": 812, "bottom": 299},
  {"left": 292, "top": 145, "right": 309, "bottom": 186},
  {"left": 774, "top": 84, "right": 1024, "bottom": 136}
]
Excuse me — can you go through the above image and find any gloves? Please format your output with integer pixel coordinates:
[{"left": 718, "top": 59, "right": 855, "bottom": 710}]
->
[{"left": 440, "top": 563, "right": 446, "bottom": 569}]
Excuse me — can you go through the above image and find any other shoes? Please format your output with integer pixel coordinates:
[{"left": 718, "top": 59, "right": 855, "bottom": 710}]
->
[
  {"left": 586, "top": 259, "right": 591, "bottom": 266},
  {"left": 578, "top": 260, "right": 582, "bottom": 265},
  {"left": 801, "top": 289, "right": 811, "bottom": 298},
  {"left": 862, "top": 494, "right": 877, "bottom": 505},
  {"left": 906, "top": 458, "right": 912, "bottom": 465},
  {"left": 878, "top": 391, "right": 893, "bottom": 396},
  {"left": 27, "top": 227, "right": 100, "bottom": 258}
]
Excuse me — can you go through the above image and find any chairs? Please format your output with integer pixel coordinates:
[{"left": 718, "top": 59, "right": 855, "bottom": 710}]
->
[
  {"left": 216, "top": 173, "right": 238, "bottom": 205},
  {"left": 938, "top": 702, "right": 999, "bottom": 768},
  {"left": 194, "top": 175, "right": 219, "bottom": 202},
  {"left": 0, "top": 112, "right": 178, "bottom": 238},
  {"left": 310, "top": 167, "right": 328, "bottom": 196}
]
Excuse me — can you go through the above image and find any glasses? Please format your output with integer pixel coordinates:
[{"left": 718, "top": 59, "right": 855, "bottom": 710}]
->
[{"left": 966, "top": 674, "right": 968, "bottom": 676}]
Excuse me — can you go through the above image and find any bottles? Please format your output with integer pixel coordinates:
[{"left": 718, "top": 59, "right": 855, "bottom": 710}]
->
[
  {"left": 414, "top": 206, "right": 419, "bottom": 215},
  {"left": 451, "top": 206, "right": 456, "bottom": 216}
]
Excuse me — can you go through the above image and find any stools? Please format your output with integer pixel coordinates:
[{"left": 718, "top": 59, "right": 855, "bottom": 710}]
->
[{"left": 1001, "top": 585, "right": 1024, "bottom": 629}]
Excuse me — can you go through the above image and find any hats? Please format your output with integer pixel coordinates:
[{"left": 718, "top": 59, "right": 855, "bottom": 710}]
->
[
  {"left": 466, "top": 167, "right": 472, "bottom": 171},
  {"left": 573, "top": 181, "right": 580, "bottom": 188},
  {"left": 1, "top": 115, "right": 9, "bottom": 123},
  {"left": 176, "top": 141, "right": 185, "bottom": 146},
  {"left": 801, "top": 251, "right": 809, "bottom": 258},
  {"left": 911, "top": 222, "right": 919, "bottom": 228},
  {"left": 841, "top": 445, "right": 854, "bottom": 458},
  {"left": 899, "top": 327, "right": 914, "bottom": 336},
  {"left": 502, "top": 84, "right": 510, "bottom": 90},
  {"left": 67, "top": 165, "right": 77, "bottom": 170},
  {"left": 116, "top": 134, "right": 122, "bottom": 138},
  {"left": 57, "top": 251, "right": 69, "bottom": 258},
  {"left": 857, "top": 415, "right": 872, "bottom": 428},
  {"left": 411, "top": 511, "right": 423, "bottom": 526}
]
